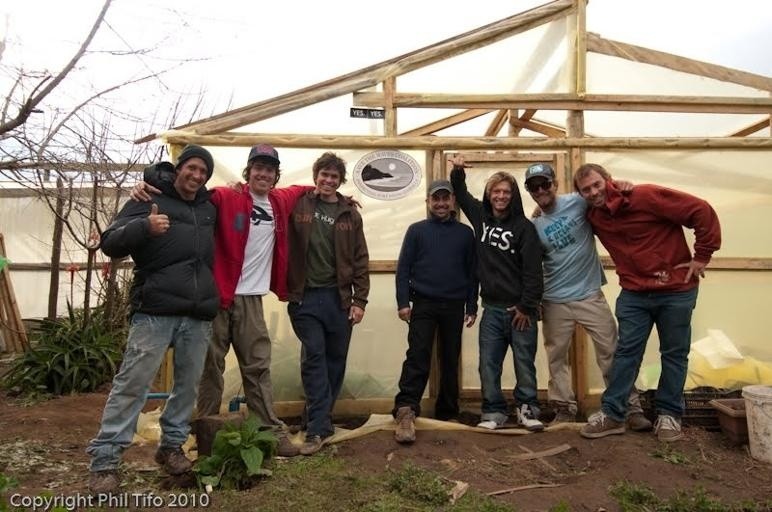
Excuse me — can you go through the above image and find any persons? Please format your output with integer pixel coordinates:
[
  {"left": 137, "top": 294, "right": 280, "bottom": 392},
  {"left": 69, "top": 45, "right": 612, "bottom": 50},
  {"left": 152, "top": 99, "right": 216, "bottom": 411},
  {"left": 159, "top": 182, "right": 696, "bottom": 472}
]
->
[
  {"left": 226, "top": 152, "right": 371, "bottom": 456},
  {"left": 129, "top": 143, "right": 363, "bottom": 457},
  {"left": 392, "top": 178, "right": 480, "bottom": 443},
  {"left": 84, "top": 143, "right": 221, "bottom": 500},
  {"left": 524, "top": 163, "right": 653, "bottom": 432},
  {"left": 447, "top": 155, "right": 548, "bottom": 435},
  {"left": 531, "top": 163, "right": 722, "bottom": 440}
]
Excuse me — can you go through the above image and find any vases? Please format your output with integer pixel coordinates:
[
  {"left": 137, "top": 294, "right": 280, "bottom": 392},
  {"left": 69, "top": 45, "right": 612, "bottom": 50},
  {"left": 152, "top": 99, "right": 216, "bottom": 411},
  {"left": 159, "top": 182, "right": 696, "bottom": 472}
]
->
[{"left": 709, "top": 398, "right": 749, "bottom": 444}]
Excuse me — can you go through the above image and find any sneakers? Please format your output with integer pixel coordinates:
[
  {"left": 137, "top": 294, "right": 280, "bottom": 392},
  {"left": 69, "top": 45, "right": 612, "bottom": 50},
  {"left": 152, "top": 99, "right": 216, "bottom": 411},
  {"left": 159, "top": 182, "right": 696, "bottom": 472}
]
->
[
  {"left": 89, "top": 427, "right": 335, "bottom": 498},
  {"left": 393, "top": 404, "right": 683, "bottom": 443}
]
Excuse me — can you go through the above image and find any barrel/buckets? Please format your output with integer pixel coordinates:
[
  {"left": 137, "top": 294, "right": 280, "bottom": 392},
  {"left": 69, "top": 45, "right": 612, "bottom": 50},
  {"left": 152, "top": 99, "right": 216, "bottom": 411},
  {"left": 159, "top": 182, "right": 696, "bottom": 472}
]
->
[{"left": 741, "top": 385, "right": 772, "bottom": 463}]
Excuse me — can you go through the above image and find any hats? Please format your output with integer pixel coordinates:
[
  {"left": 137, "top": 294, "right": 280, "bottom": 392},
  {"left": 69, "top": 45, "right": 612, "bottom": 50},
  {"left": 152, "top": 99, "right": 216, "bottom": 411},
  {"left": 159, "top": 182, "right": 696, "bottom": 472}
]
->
[
  {"left": 248, "top": 143, "right": 280, "bottom": 166},
  {"left": 173, "top": 144, "right": 213, "bottom": 180},
  {"left": 427, "top": 180, "right": 454, "bottom": 196},
  {"left": 525, "top": 163, "right": 555, "bottom": 184}
]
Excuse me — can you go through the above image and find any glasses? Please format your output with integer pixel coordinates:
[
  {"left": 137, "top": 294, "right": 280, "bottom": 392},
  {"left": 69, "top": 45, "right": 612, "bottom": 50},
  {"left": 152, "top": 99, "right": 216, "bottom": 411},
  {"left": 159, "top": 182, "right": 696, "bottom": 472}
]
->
[{"left": 527, "top": 180, "right": 551, "bottom": 192}]
